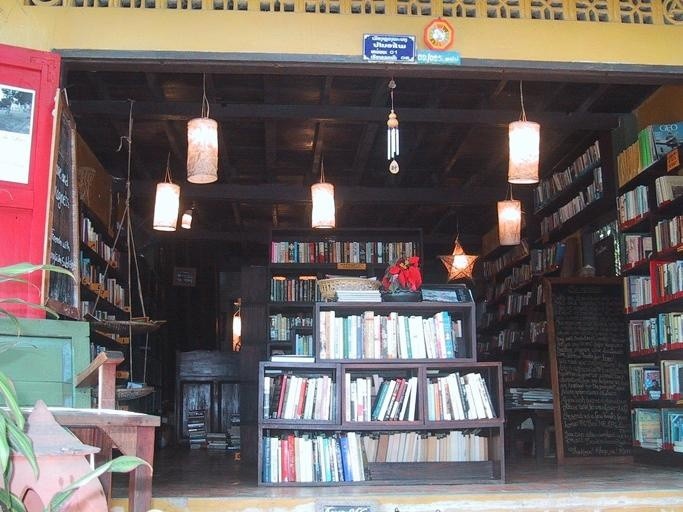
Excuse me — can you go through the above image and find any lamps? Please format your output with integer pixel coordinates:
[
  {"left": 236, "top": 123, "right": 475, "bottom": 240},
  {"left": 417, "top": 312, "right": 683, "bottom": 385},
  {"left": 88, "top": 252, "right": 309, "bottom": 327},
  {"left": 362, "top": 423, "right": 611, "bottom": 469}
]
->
[
  {"left": 152, "top": 152, "right": 180, "bottom": 231},
  {"left": 508, "top": 78, "right": 541, "bottom": 183},
  {"left": 309, "top": 153, "right": 336, "bottom": 229},
  {"left": 232, "top": 299, "right": 241, "bottom": 336},
  {"left": 187, "top": 74, "right": 221, "bottom": 185},
  {"left": 181, "top": 209, "right": 191, "bottom": 228},
  {"left": 498, "top": 172, "right": 521, "bottom": 248}
]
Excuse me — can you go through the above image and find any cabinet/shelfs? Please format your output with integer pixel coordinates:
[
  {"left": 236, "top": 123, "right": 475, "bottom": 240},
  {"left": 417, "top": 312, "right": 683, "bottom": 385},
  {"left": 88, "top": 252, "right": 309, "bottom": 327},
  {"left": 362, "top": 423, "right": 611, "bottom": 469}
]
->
[
  {"left": 439, "top": 211, "right": 478, "bottom": 285},
  {"left": 480, "top": 116, "right": 616, "bottom": 457},
  {"left": 77, "top": 206, "right": 132, "bottom": 366},
  {"left": 256, "top": 362, "right": 505, "bottom": 484},
  {"left": 312, "top": 300, "right": 476, "bottom": 361},
  {"left": 266, "top": 228, "right": 423, "bottom": 359},
  {"left": 618, "top": 144, "right": 683, "bottom": 464}
]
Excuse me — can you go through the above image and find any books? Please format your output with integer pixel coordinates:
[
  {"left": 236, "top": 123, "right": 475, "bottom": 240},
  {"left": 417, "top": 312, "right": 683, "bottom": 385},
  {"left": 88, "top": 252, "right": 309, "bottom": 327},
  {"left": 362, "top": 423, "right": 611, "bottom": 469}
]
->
[
  {"left": 187, "top": 410, "right": 242, "bottom": 451},
  {"left": 473, "top": 122, "right": 682, "bottom": 458},
  {"left": 81, "top": 217, "right": 125, "bottom": 361},
  {"left": 263, "top": 236, "right": 497, "bottom": 482}
]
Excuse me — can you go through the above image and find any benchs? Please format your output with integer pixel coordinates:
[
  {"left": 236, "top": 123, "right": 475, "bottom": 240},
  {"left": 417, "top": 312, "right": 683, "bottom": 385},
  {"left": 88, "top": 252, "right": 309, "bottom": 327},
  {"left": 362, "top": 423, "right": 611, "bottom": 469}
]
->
[{"left": 1, "top": 318, "right": 165, "bottom": 509}]
